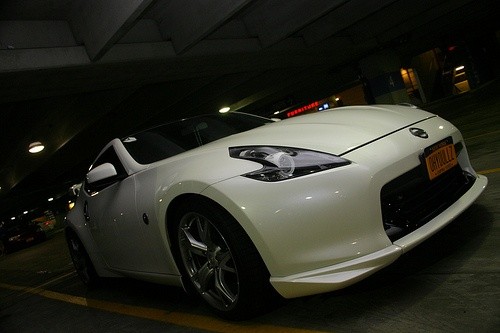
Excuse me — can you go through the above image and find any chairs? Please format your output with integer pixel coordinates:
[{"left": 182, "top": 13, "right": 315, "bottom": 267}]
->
[{"left": 130, "top": 139, "right": 162, "bottom": 163}]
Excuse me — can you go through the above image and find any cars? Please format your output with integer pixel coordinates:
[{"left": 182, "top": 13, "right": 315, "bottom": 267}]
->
[
  {"left": 0, "top": 208, "right": 56, "bottom": 253},
  {"left": 62, "top": 103, "right": 490, "bottom": 321}
]
